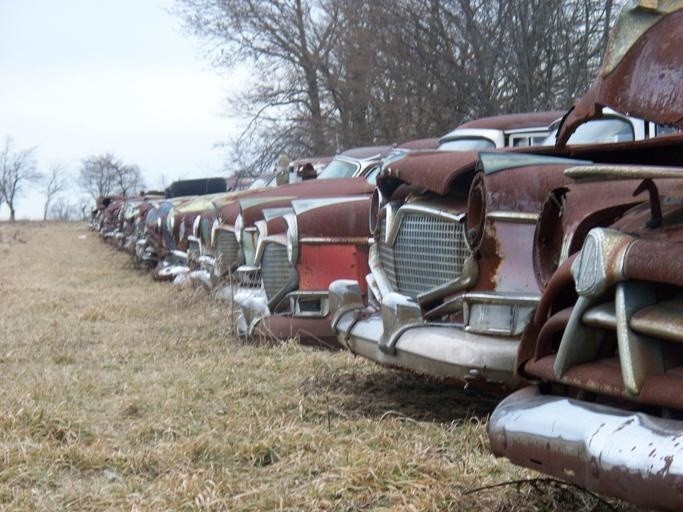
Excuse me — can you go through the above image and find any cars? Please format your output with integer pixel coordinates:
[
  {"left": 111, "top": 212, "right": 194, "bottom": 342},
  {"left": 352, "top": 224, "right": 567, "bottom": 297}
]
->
[
  {"left": 330, "top": 107, "right": 683, "bottom": 396},
  {"left": 88, "top": 116, "right": 565, "bottom": 339},
  {"left": 490, "top": 0, "right": 683, "bottom": 512}
]
